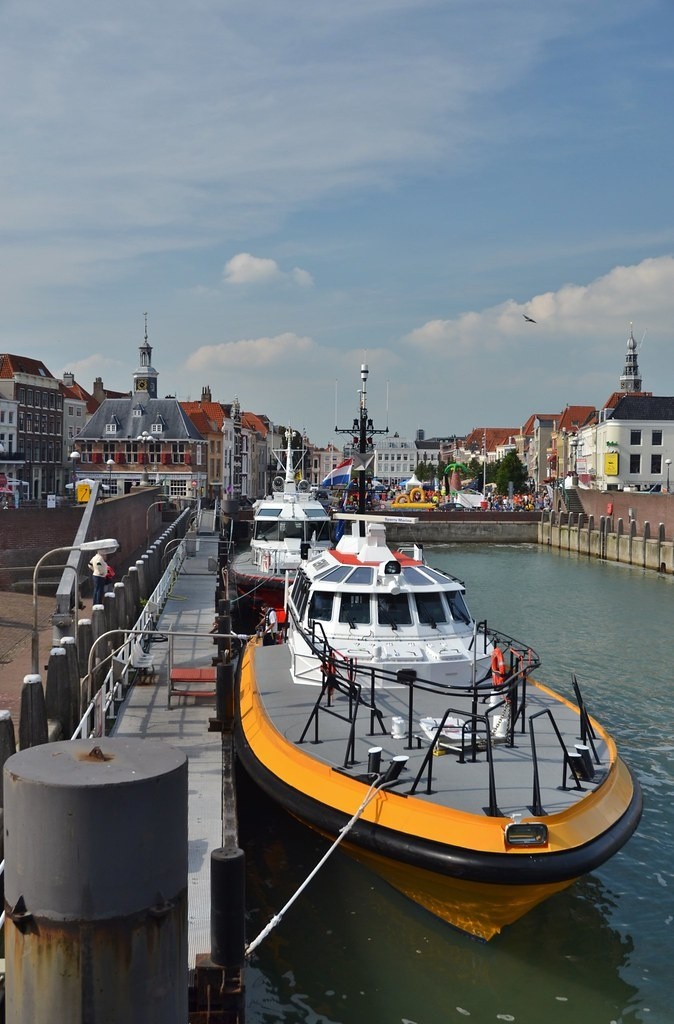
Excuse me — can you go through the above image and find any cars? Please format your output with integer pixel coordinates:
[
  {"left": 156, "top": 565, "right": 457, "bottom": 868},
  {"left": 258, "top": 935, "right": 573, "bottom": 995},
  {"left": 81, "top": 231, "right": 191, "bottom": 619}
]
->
[{"left": 434, "top": 503, "right": 474, "bottom": 513}]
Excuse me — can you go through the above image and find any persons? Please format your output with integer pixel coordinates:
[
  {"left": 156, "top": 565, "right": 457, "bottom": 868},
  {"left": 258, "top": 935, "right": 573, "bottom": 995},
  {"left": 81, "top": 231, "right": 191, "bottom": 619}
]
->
[
  {"left": 351, "top": 486, "right": 454, "bottom": 514},
  {"left": 256, "top": 603, "right": 278, "bottom": 646},
  {"left": 489, "top": 492, "right": 550, "bottom": 511},
  {"left": 88, "top": 551, "right": 113, "bottom": 606}
]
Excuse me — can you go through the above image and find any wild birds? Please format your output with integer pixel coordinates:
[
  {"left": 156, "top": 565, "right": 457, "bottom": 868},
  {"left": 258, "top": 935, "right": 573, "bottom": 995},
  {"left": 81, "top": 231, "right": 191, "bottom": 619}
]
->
[{"left": 522, "top": 314, "right": 537, "bottom": 324}]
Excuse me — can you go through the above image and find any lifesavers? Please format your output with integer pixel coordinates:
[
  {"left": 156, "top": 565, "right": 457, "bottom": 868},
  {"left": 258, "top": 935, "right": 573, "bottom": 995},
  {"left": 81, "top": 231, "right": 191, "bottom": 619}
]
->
[{"left": 490, "top": 648, "right": 506, "bottom": 688}]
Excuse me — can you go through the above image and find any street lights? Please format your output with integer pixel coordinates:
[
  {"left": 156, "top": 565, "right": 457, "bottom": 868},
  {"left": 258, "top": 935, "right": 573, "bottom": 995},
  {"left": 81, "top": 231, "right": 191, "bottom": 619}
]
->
[
  {"left": 69, "top": 451, "right": 81, "bottom": 506},
  {"left": 137, "top": 431, "right": 154, "bottom": 485},
  {"left": 29, "top": 537, "right": 120, "bottom": 678},
  {"left": 105, "top": 458, "right": 116, "bottom": 499},
  {"left": 145, "top": 501, "right": 167, "bottom": 552},
  {"left": 600, "top": 490, "right": 615, "bottom": 534},
  {"left": 664, "top": 458, "right": 673, "bottom": 493}
]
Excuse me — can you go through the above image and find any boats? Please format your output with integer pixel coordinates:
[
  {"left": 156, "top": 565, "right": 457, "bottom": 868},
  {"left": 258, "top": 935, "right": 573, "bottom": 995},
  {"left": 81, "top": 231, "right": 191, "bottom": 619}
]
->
[{"left": 230, "top": 365, "right": 648, "bottom": 941}]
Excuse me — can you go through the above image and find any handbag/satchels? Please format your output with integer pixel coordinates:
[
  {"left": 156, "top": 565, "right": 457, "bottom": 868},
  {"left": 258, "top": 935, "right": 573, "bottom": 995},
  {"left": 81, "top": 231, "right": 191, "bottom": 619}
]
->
[{"left": 106, "top": 565, "right": 116, "bottom": 579}]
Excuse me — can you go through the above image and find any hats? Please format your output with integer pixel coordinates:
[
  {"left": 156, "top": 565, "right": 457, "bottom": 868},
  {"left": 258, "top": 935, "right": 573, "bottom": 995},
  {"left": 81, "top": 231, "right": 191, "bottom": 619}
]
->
[{"left": 487, "top": 497, "right": 492, "bottom": 500}]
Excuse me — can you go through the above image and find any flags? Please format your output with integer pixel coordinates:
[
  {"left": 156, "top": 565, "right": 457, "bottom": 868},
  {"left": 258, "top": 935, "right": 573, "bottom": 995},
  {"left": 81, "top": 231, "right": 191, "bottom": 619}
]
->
[{"left": 323, "top": 457, "right": 351, "bottom": 485}]
